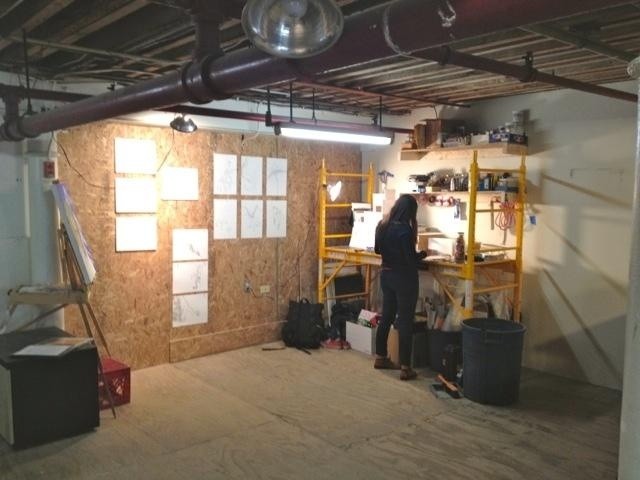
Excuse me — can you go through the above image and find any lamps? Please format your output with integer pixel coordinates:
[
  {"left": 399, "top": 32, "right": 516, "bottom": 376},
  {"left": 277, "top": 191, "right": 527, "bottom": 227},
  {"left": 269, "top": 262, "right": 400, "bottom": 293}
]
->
[
  {"left": 167, "top": 112, "right": 198, "bottom": 135},
  {"left": 241, "top": 0, "right": 346, "bottom": 60},
  {"left": 321, "top": 179, "right": 342, "bottom": 203},
  {"left": 264, "top": 80, "right": 395, "bottom": 146}
]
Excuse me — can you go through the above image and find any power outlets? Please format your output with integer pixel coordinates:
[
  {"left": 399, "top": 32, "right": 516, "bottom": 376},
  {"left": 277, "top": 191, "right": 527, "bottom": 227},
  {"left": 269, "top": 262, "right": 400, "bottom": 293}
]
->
[{"left": 44, "top": 160, "right": 53, "bottom": 177}]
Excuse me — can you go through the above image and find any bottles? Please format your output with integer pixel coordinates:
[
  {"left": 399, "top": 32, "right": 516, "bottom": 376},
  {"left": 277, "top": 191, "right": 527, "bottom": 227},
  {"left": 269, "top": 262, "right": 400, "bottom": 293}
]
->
[
  {"left": 498, "top": 108, "right": 526, "bottom": 136},
  {"left": 454, "top": 230, "right": 465, "bottom": 264}
]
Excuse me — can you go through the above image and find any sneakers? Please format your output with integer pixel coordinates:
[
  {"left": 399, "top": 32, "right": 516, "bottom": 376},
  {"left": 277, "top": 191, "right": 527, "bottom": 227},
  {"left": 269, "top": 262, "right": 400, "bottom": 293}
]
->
[
  {"left": 320, "top": 338, "right": 349, "bottom": 350},
  {"left": 374, "top": 358, "right": 400, "bottom": 370},
  {"left": 401, "top": 368, "right": 417, "bottom": 379}
]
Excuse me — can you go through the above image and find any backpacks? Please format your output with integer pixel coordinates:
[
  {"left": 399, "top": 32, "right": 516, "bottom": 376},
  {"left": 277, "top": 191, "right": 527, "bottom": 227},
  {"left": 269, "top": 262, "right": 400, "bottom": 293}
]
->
[
  {"left": 330, "top": 303, "right": 358, "bottom": 338},
  {"left": 281, "top": 298, "right": 325, "bottom": 356}
]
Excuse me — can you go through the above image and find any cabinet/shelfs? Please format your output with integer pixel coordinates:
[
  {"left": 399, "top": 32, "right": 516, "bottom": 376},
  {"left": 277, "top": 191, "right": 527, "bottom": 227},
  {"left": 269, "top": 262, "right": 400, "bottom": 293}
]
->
[
  {"left": 0, "top": 326, "right": 100, "bottom": 450},
  {"left": 316, "top": 149, "right": 526, "bottom": 387}
]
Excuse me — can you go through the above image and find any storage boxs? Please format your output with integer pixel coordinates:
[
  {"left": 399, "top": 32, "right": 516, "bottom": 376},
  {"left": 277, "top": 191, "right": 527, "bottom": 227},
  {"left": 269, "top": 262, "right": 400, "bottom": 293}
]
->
[{"left": 343, "top": 302, "right": 429, "bottom": 370}]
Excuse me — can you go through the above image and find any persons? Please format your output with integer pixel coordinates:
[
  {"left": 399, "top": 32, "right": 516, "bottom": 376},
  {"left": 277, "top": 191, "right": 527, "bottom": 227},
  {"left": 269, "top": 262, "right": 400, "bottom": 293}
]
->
[{"left": 374, "top": 193, "right": 439, "bottom": 381}]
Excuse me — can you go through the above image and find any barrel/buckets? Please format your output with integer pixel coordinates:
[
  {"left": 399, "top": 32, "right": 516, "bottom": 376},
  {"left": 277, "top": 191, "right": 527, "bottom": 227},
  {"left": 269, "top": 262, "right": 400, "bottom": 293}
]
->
[{"left": 429, "top": 325, "right": 463, "bottom": 373}]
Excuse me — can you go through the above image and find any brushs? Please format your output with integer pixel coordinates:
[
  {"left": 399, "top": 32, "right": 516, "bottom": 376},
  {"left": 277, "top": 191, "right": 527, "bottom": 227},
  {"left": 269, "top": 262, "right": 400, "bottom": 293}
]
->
[{"left": 438, "top": 375, "right": 459, "bottom": 398}]
